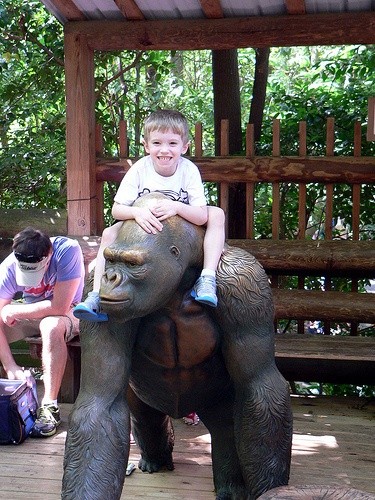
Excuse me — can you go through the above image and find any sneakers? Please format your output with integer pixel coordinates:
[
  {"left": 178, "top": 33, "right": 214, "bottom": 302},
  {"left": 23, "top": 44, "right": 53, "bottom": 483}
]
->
[
  {"left": 72, "top": 292, "right": 108, "bottom": 322},
  {"left": 191, "top": 274, "right": 218, "bottom": 307},
  {"left": 29, "top": 402, "right": 61, "bottom": 437}
]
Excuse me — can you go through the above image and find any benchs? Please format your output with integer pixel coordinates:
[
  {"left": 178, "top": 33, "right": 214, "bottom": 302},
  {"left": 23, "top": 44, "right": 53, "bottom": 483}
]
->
[{"left": 20, "top": 231, "right": 375, "bottom": 404}]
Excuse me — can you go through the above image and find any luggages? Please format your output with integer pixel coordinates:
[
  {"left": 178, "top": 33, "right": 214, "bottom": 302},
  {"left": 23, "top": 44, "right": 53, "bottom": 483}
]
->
[{"left": 1, "top": 379, "right": 39, "bottom": 448}]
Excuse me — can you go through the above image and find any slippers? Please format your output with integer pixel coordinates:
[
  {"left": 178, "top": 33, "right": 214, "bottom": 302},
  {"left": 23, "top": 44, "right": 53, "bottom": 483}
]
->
[{"left": 183, "top": 411, "right": 200, "bottom": 426}]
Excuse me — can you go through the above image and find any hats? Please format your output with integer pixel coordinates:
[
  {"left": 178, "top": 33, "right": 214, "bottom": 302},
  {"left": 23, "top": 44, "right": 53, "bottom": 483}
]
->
[{"left": 13, "top": 250, "right": 54, "bottom": 287}]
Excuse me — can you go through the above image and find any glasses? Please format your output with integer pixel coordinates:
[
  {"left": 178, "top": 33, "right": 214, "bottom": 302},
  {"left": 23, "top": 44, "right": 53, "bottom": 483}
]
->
[{"left": 14, "top": 251, "right": 49, "bottom": 262}]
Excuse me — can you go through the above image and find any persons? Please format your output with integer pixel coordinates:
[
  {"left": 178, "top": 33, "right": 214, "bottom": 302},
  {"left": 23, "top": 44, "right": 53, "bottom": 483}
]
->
[
  {"left": 0, "top": 226, "right": 87, "bottom": 438},
  {"left": 74, "top": 111, "right": 225, "bottom": 323}
]
